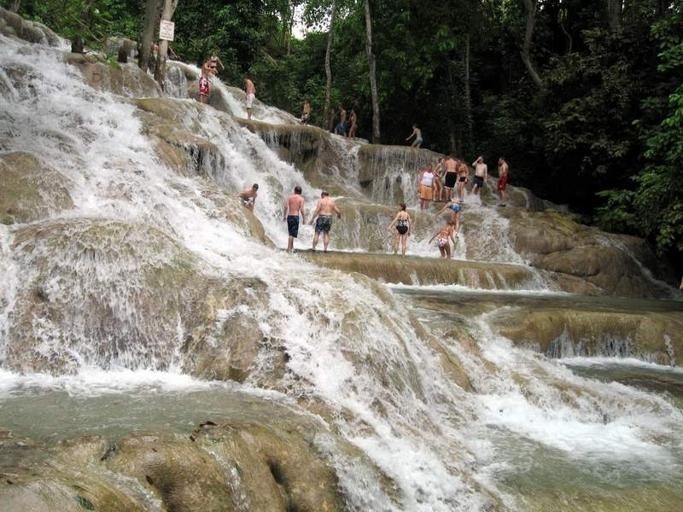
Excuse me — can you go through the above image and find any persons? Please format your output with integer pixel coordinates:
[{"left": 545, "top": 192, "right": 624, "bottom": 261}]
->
[
  {"left": 346, "top": 107, "right": 357, "bottom": 141},
  {"left": 428, "top": 216, "right": 455, "bottom": 260},
  {"left": 282, "top": 186, "right": 305, "bottom": 252},
  {"left": 197, "top": 56, "right": 218, "bottom": 103},
  {"left": 470, "top": 156, "right": 488, "bottom": 198},
  {"left": 496, "top": 156, "right": 509, "bottom": 207},
  {"left": 298, "top": 98, "right": 311, "bottom": 126},
  {"left": 334, "top": 104, "right": 346, "bottom": 137},
  {"left": 243, "top": 75, "right": 257, "bottom": 119},
  {"left": 238, "top": 183, "right": 258, "bottom": 210},
  {"left": 389, "top": 202, "right": 411, "bottom": 256},
  {"left": 415, "top": 153, "right": 469, "bottom": 211},
  {"left": 307, "top": 191, "right": 341, "bottom": 252},
  {"left": 209, "top": 51, "right": 225, "bottom": 75},
  {"left": 404, "top": 122, "right": 423, "bottom": 149},
  {"left": 430, "top": 199, "right": 461, "bottom": 234}
]
